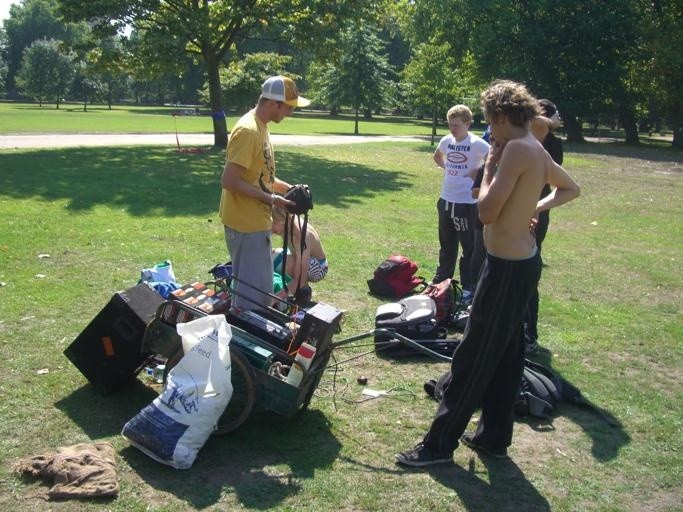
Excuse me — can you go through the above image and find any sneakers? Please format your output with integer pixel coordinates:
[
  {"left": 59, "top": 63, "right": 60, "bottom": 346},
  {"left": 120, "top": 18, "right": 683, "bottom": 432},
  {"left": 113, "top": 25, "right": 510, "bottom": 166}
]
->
[
  {"left": 524, "top": 339, "right": 541, "bottom": 357},
  {"left": 393, "top": 441, "right": 460, "bottom": 467},
  {"left": 459, "top": 429, "right": 508, "bottom": 459}
]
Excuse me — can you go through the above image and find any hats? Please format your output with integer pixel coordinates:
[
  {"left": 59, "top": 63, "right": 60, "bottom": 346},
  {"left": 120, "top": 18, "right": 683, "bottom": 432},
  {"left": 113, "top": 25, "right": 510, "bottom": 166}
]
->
[{"left": 259, "top": 74, "right": 312, "bottom": 108}]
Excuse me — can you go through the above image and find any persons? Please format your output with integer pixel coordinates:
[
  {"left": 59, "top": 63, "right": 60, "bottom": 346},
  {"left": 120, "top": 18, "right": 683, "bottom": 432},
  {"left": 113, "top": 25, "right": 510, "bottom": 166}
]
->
[
  {"left": 271, "top": 204, "right": 329, "bottom": 305},
  {"left": 521, "top": 97, "right": 564, "bottom": 342},
  {"left": 430, "top": 104, "right": 490, "bottom": 301},
  {"left": 394, "top": 78, "right": 581, "bottom": 468},
  {"left": 216, "top": 73, "right": 312, "bottom": 308}
]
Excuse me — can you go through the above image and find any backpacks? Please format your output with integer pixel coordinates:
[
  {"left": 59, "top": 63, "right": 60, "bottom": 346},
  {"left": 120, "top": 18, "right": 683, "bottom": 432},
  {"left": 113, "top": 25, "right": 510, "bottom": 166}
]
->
[
  {"left": 420, "top": 276, "right": 464, "bottom": 325},
  {"left": 374, "top": 292, "right": 449, "bottom": 358},
  {"left": 424, "top": 354, "right": 581, "bottom": 433}
]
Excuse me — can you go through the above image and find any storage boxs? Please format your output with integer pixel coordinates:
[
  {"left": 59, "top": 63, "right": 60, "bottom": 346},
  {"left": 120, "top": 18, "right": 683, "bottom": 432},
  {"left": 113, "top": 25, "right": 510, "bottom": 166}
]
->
[
  {"left": 158, "top": 282, "right": 228, "bottom": 326},
  {"left": 219, "top": 311, "right": 292, "bottom": 376},
  {"left": 290, "top": 299, "right": 342, "bottom": 360}
]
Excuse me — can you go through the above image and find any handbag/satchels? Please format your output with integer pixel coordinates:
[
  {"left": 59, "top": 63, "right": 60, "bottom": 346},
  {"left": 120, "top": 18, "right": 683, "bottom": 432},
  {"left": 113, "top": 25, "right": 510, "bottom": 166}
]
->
[
  {"left": 136, "top": 259, "right": 177, "bottom": 286},
  {"left": 367, "top": 254, "right": 430, "bottom": 301}
]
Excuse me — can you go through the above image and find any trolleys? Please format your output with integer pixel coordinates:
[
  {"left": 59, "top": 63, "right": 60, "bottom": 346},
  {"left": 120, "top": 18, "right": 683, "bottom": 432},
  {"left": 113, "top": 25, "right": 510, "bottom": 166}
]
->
[{"left": 139, "top": 266, "right": 403, "bottom": 436}]
